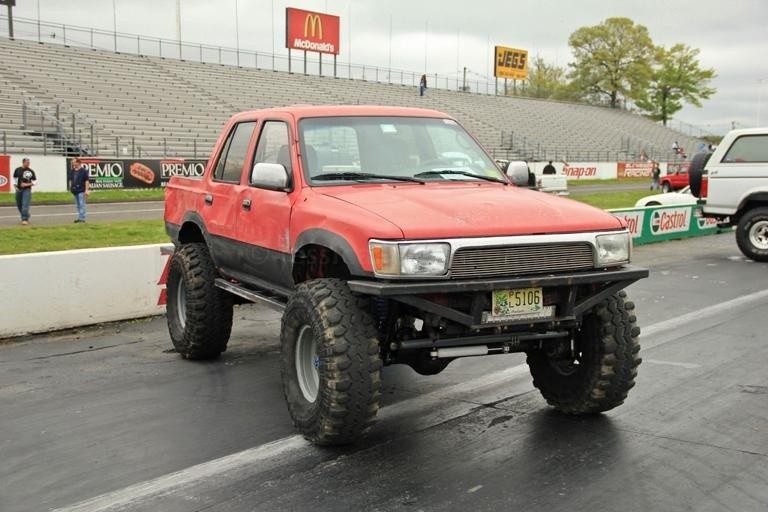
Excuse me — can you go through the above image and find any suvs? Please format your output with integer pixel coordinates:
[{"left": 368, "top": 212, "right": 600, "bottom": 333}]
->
[{"left": 688, "top": 127, "right": 768, "bottom": 261}]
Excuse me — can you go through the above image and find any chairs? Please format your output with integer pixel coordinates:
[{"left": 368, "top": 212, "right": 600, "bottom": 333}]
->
[{"left": 277, "top": 145, "right": 324, "bottom": 178}]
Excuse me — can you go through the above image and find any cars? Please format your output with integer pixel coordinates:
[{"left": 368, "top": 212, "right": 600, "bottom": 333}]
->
[{"left": 634, "top": 183, "right": 700, "bottom": 208}]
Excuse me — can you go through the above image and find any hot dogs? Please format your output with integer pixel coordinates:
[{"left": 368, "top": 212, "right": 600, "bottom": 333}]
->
[{"left": 130, "top": 164, "right": 155, "bottom": 184}]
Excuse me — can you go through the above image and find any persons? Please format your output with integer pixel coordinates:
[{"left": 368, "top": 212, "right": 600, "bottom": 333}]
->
[
  {"left": 672, "top": 141, "right": 680, "bottom": 154},
  {"left": 13, "top": 158, "right": 37, "bottom": 225},
  {"left": 640, "top": 149, "right": 648, "bottom": 161},
  {"left": 69, "top": 158, "right": 89, "bottom": 222},
  {"left": 651, "top": 163, "right": 660, "bottom": 190},
  {"left": 543, "top": 161, "right": 555, "bottom": 174},
  {"left": 420, "top": 78, "right": 424, "bottom": 96}
]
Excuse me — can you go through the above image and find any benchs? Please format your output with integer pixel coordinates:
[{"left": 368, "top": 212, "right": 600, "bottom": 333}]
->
[{"left": 1, "top": 38, "right": 719, "bottom": 159}]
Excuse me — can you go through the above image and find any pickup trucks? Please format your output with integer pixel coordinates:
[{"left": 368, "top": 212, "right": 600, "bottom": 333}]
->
[
  {"left": 163, "top": 102, "right": 651, "bottom": 447},
  {"left": 658, "top": 164, "right": 690, "bottom": 193}
]
